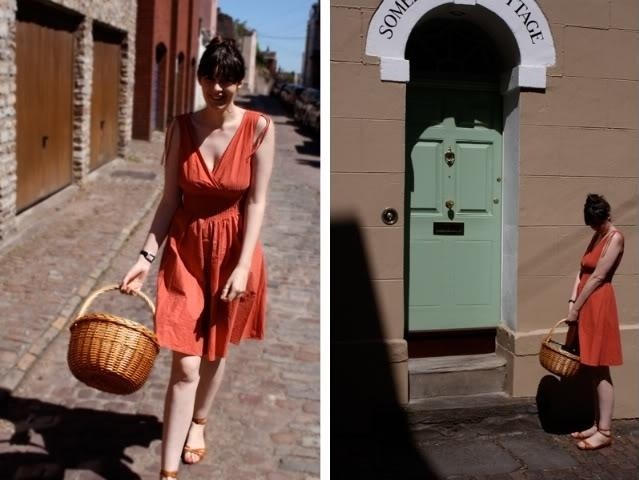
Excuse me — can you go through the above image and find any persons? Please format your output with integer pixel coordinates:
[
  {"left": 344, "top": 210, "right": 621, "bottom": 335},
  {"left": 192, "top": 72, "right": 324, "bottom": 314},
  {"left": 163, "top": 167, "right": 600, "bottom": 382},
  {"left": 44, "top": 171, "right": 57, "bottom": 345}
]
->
[
  {"left": 568, "top": 194, "right": 623, "bottom": 450},
  {"left": 120, "top": 36, "right": 276, "bottom": 479}
]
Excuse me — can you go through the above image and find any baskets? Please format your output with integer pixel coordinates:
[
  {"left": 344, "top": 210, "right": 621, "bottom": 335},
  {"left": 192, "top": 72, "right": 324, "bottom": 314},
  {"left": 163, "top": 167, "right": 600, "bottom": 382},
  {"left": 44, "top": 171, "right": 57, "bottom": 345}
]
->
[
  {"left": 68, "top": 285, "right": 161, "bottom": 394},
  {"left": 540, "top": 317, "right": 580, "bottom": 379}
]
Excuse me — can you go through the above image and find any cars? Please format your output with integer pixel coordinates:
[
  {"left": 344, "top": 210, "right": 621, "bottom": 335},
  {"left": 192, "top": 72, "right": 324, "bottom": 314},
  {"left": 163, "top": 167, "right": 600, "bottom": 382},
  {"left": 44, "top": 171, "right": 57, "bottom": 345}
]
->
[{"left": 271, "top": 80, "right": 320, "bottom": 130}]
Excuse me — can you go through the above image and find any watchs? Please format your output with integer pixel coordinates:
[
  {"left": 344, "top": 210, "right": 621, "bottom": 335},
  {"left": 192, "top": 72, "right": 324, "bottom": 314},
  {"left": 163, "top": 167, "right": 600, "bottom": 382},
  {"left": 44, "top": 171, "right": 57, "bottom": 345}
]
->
[{"left": 141, "top": 250, "right": 155, "bottom": 262}]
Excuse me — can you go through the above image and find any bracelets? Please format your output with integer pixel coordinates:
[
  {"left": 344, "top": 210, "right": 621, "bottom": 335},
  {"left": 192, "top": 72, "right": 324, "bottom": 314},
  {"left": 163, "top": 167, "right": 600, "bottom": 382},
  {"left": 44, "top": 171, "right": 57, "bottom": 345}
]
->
[{"left": 569, "top": 300, "right": 575, "bottom": 303}]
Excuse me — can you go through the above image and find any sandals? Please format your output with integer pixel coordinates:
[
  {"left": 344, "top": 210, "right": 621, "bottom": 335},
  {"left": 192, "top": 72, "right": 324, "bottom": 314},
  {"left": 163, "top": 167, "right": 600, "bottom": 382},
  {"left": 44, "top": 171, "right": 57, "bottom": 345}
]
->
[
  {"left": 183, "top": 418, "right": 206, "bottom": 464},
  {"left": 571, "top": 423, "right": 611, "bottom": 450}
]
